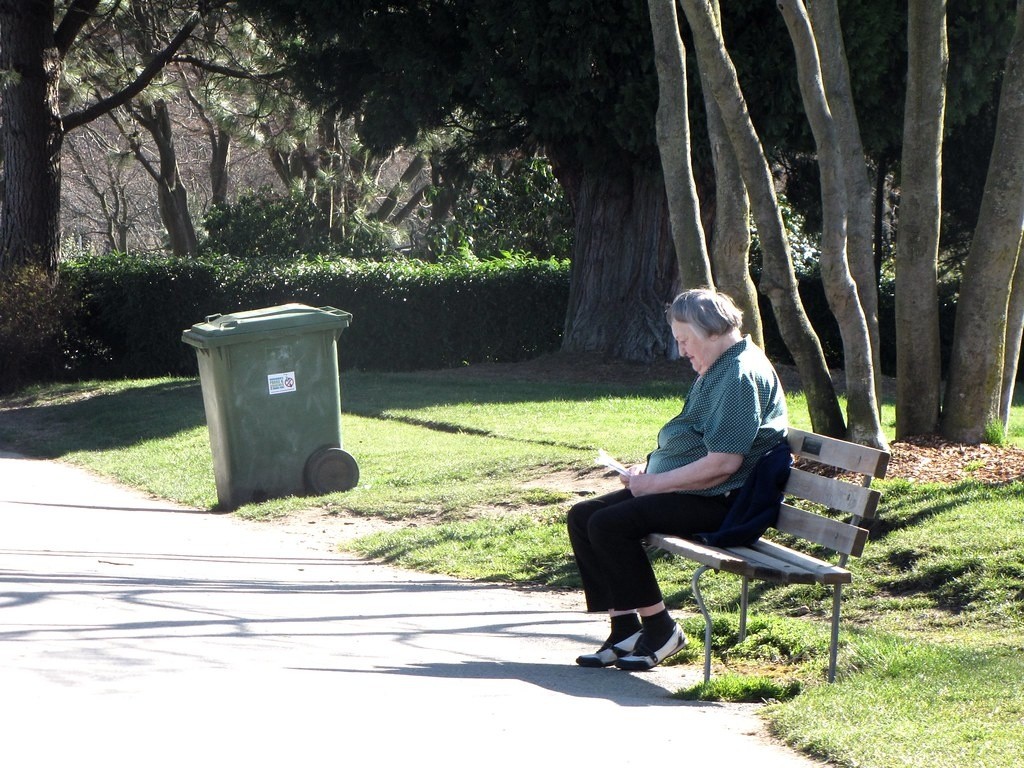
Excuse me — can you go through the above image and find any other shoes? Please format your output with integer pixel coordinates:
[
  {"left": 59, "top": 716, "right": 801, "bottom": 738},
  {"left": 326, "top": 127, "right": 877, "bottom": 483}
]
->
[
  {"left": 614, "top": 621, "right": 690, "bottom": 671},
  {"left": 576, "top": 627, "right": 644, "bottom": 668}
]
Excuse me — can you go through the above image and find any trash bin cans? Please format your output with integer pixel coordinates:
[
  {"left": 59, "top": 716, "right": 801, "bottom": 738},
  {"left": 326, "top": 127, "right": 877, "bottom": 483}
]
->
[{"left": 181, "top": 302, "right": 360, "bottom": 508}]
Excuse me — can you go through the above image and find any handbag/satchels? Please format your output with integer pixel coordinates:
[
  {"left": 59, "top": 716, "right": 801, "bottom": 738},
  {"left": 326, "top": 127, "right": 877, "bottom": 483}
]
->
[{"left": 691, "top": 442, "right": 792, "bottom": 547}]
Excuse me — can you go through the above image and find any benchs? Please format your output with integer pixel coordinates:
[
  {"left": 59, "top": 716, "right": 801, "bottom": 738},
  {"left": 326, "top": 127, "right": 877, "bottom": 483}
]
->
[{"left": 645, "top": 425, "right": 891, "bottom": 684}]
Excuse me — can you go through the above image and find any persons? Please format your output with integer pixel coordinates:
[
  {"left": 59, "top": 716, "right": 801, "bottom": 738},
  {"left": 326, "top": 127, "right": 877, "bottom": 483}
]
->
[{"left": 567, "top": 289, "right": 790, "bottom": 670}]
